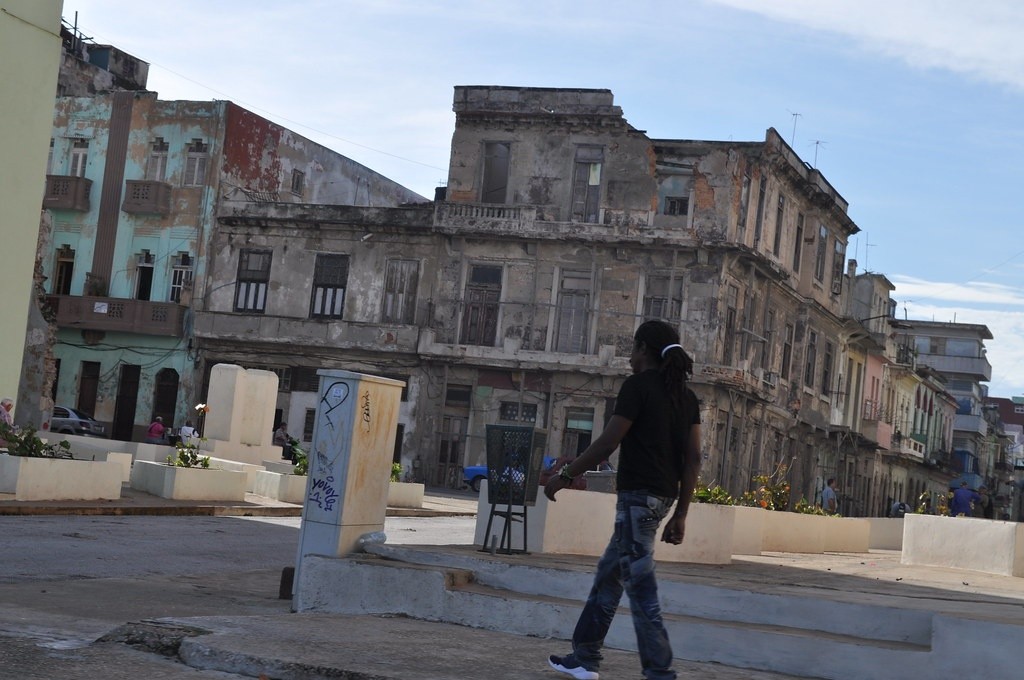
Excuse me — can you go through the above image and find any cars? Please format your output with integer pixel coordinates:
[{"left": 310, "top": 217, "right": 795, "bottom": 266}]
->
[{"left": 50, "top": 406, "right": 107, "bottom": 438}]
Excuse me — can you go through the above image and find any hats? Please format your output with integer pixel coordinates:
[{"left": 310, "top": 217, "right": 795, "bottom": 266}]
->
[{"left": 156, "top": 416, "right": 163, "bottom": 422}]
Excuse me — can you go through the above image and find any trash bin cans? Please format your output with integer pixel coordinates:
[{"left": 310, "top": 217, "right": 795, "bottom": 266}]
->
[{"left": 583, "top": 470, "right": 617, "bottom": 493}]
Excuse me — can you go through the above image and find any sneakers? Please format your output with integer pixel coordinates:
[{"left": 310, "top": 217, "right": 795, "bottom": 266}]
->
[{"left": 548, "top": 652, "right": 600, "bottom": 680}]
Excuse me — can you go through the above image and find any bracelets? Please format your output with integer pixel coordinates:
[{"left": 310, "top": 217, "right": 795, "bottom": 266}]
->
[{"left": 558, "top": 464, "right": 576, "bottom": 480}]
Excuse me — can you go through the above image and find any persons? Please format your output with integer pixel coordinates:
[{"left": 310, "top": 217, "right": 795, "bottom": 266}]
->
[
  {"left": 543, "top": 321, "right": 703, "bottom": 678},
  {"left": 951, "top": 480, "right": 980, "bottom": 516},
  {"left": 274, "top": 422, "right": 294, "bottom": 448},
  {"left": 822, "top": 479, "right": 838, "bottom": 514},
  {"left": 601, "top": 457, "right": 616, "bottom": 471},
  {"left": 974, "top": 483, "right": 994, "bottom": 519},
  {"left": 144, "top": 417, "right": 169, "bottom": 444},
  {"left": 890, "top": 503, "right": 910, "bottom": 517},
  {"left": 0, "top": 398, "right": 18, "bottom": 448},
  {"left": 924, "top": 498, "right": 936, "bottom": 515},
  {"left": 180, "top": 421, "right": 200, "bottom": 447}
]
